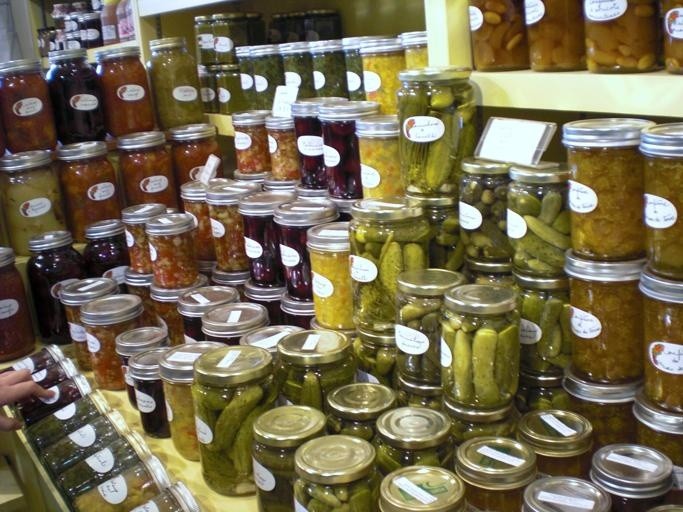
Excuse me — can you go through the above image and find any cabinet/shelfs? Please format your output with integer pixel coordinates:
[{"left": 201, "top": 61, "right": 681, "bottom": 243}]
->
[{"left": 0, "top": 0, "right": 683, "bottom": 512}]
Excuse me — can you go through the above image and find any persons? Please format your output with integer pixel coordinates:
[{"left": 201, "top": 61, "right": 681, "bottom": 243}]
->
[{"left": 0, "top": 369, "right": 55, "bottom": 431}]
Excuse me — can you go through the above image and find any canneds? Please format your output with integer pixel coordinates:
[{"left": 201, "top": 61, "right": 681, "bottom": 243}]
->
[{"left": 0, "top": 0, "right": 683, "bottom": 512}]
